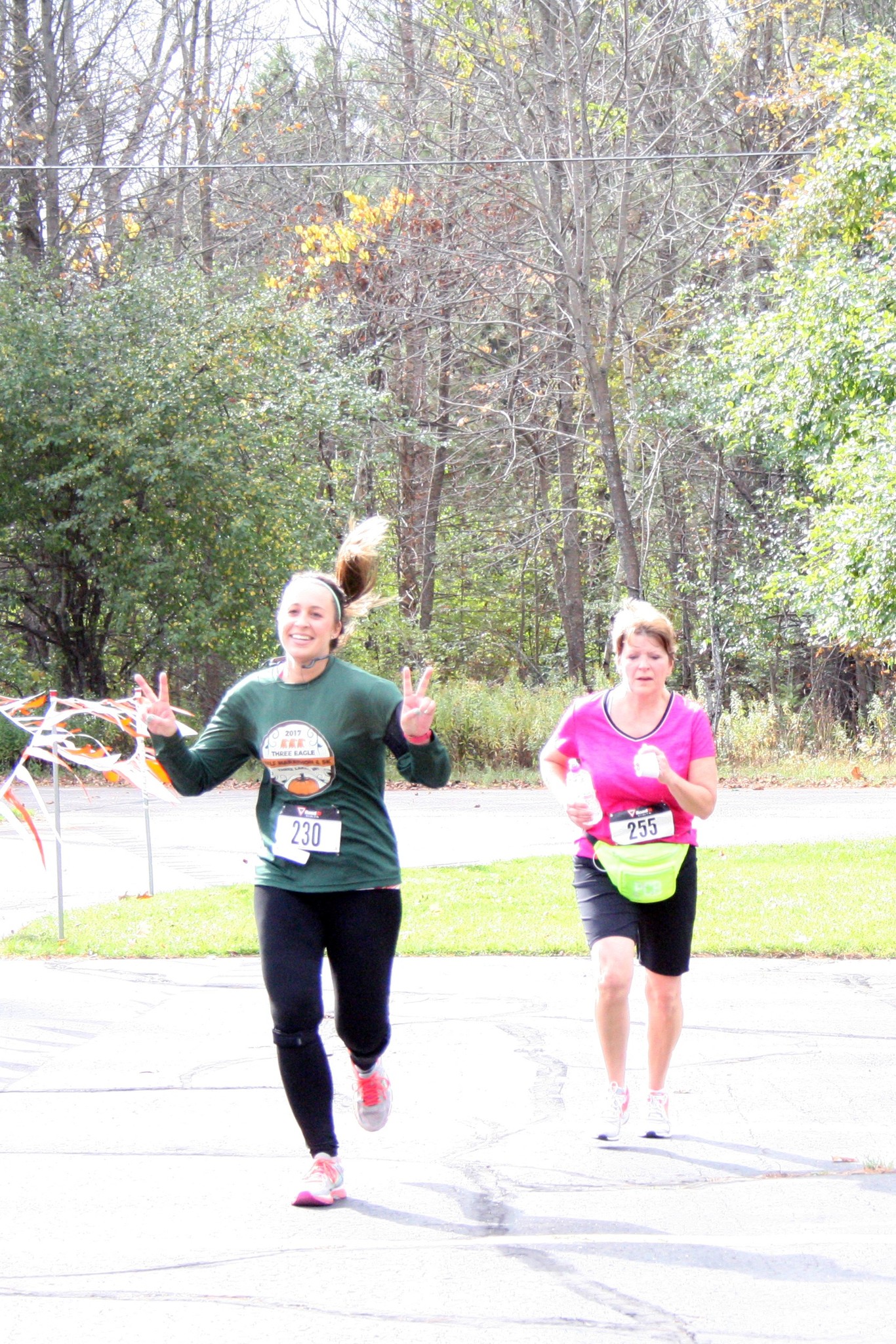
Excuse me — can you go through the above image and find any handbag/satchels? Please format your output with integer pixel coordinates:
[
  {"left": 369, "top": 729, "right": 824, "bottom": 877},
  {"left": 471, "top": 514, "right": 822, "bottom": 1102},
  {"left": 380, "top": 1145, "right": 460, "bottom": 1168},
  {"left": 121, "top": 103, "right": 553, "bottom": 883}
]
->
[{"left": 585, "top": 829, "right": 690, "bottom": 903}]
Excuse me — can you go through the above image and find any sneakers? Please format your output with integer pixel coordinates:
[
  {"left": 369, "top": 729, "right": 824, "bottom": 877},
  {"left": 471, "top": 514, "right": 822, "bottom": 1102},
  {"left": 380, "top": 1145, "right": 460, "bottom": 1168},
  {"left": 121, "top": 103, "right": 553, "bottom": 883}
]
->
[
  {"left": 353, "top": 1060, "right": 390, "bottom": 1131},
  {"left": 292, "top": 1150, "right": 346, "bottom": 1207},
  {"left": 640, "top": 1091, "right": 668, "bottom": 1139},
  {"left": 594, "top": 1082, "right": 630, "bottom": 1141}
]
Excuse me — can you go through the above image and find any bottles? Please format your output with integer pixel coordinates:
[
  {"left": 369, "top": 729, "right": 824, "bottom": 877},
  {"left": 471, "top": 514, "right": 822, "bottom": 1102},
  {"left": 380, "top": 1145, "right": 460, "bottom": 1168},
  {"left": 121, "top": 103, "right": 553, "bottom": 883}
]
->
[{"left": 567, "top": 758, "right": 603, "bottom": 825}]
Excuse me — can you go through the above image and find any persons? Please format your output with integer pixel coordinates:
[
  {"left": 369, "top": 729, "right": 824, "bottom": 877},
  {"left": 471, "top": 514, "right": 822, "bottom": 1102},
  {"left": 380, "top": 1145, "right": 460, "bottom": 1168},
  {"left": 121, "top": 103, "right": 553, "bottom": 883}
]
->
[
  {"left": 540, "top": 598, "right": 717, "bottom": 1142},
  {"left": 132, "top": 517, "right": 452, "bottom": 1207}
]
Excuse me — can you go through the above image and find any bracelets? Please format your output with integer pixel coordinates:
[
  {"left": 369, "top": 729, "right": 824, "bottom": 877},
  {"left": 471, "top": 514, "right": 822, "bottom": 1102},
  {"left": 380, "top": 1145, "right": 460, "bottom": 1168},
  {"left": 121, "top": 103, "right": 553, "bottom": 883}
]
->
[{"left": 403, "top": 730, "right": 432, "bottom": 743}]
